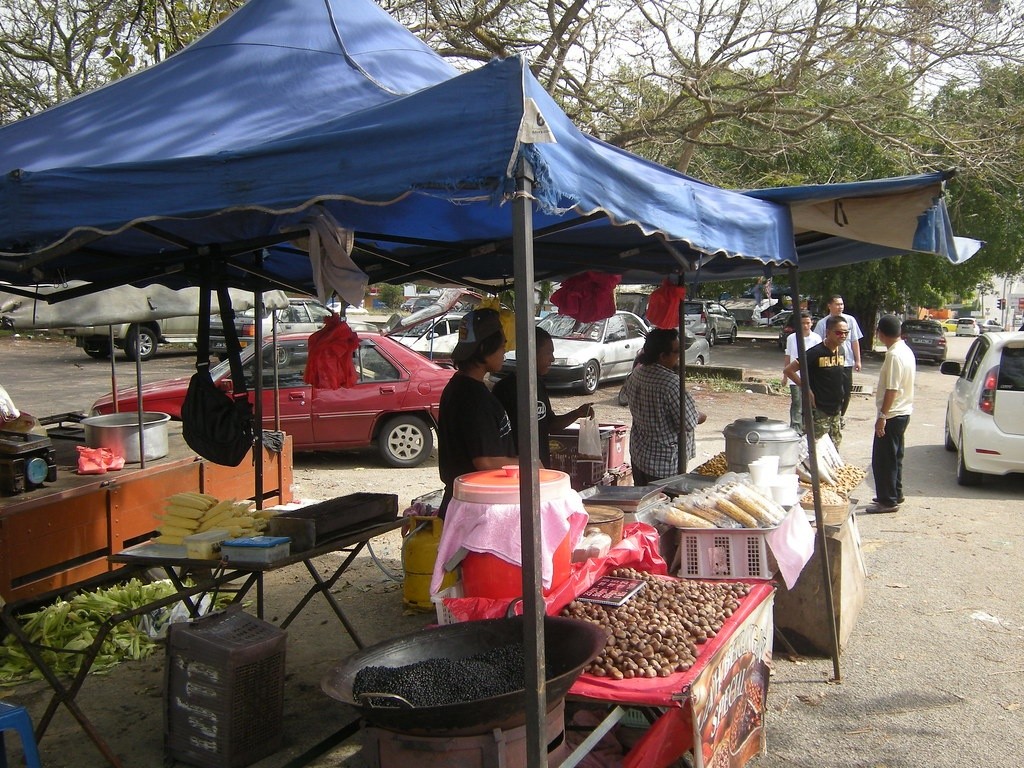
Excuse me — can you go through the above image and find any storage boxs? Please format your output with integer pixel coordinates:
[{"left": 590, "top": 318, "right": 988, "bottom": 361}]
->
[
  {"left": 184, "top": 529, "right": 232, "bottom": 560},
  {"left": 220, "top": 534, "right": 293, "bottom": 562},
  {"left": 549, "top": 429, "right": 613, "bottom": 481},
  {"left": 607, "top": 703, "right": 669, "bottom": 729},
  {"left": 798, "top": 488, "right": 809, "bottom": 500},
  {"left": 570, "top": 423, "right": 628, "bottom": 470},
  {"left": 160, "top": 602, "right": 289, "bottom": 768},
  {"left": 676, "top": 504, "right": 796, "bottom": 579}
]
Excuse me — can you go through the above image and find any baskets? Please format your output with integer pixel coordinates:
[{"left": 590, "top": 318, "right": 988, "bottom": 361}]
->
[{"left": 798, "top": 494, "right": 850, "bottom": 525}]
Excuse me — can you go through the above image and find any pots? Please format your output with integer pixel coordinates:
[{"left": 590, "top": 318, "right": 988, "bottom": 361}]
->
[
  {"left": 320, "top": 597, "right": 608, "bottom": 738},
  {"left": 723, "top": 415, "right": 800, "bottom": 477}
]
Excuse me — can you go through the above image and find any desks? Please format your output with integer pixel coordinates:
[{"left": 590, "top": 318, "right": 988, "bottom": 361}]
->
[
  {"left": 698, "top": 571, "right": 799, "bottom": 661},
  {"left": 107, "top": 499, "right": 410, "bottom": 650}
]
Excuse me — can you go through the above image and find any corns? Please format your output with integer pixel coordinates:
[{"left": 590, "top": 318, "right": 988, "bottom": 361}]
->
[
  {"left": 655, "top": 484, "right": 788, "bottom": 528},
  {"left": 150, "top": 491, "right": 278, "bottom": 546}
]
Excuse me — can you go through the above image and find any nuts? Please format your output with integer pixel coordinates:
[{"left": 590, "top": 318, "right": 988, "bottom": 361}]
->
[{"left": 553, "top": 566, "right": 752, "bottom": 680}]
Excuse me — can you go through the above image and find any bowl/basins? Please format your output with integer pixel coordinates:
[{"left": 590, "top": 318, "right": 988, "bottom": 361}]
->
[{"left": 82, "top": 410, "right": 171, "bottom": 465}]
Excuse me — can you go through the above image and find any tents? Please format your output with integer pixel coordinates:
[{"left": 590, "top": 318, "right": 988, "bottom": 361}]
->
[{"left": 0, "top": 0, "right": 988, "bottom": 768}]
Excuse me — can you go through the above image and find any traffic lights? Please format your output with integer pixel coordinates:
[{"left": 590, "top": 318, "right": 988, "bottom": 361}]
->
[
  {"left": 997, "top": 299, "right": 1001, "bottom": 309},
  {"left": 1001, "top": 299, "right": 1006, "bottom": 310}
]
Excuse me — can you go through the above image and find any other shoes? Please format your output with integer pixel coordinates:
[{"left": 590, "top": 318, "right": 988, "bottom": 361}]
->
[
  {"left": 872, "top": 496, "right": 906, "bottom": 503},
  {"left": 864, "top": 503, "right": 899, "bottom": 513}
]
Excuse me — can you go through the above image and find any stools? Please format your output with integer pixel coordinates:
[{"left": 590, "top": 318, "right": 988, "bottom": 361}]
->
[{"left": 0, "top": 700, "right": 42, "bottom": 768}]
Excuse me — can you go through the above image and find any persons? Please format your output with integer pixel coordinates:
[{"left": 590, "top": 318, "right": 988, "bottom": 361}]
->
[
  {"left": 618, "top": 327, "right": 708, "bottom": 486},
  {"left": 437, "top": 308, "right": 545, "bottom": 531},
  {"left": 783, "top": 315, "right": 850, "bottom": 455},
  {"left": 491, "top": 326, "right": 595, "bottom": 469},
  {"left": 928, "top": 313, "right": 934, "bottom": 321},
  {"left": 865, "top": 314, "right": 917, "bottom": 514},
  {"left": 782, "top": 311, "right": 822, "bottom": 437},
  {"left": 1018, "top": 310, "right": 1024, "bottom": 331},
  {"left": 813, "top": 294, "right": 863, "bottom": 430},
  {"left": 922, "top": 315, "right": 928, "bottom": 320},
  {"left": 994, "top": 317, "right": 998, "bottom": 324}
]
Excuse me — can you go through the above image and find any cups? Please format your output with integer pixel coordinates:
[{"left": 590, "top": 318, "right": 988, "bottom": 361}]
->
[{"left": 747, "top": 454, "right": 800, "bottom": 506}]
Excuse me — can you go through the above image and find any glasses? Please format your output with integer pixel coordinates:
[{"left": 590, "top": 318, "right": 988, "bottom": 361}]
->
[{"left": 831, "top": 330, "right": 849, "bottom": 336}]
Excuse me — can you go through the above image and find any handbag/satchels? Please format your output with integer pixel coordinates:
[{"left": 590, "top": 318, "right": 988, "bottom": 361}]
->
[
  {"left": 180, "top": 370, "right": 250, "bottom": 467},
  {"left": 578, "top": 404, "right": 602, "bottom": 460}
]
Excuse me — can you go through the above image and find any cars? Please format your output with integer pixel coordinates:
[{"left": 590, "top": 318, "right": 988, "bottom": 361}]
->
[
  {"left": 33, "top": 315, "right": 199, "bottom": 362},
  {"left": 649, "top": 325, "right": 711, "bottom": 365},
  {"left": 758, "top": 311, "right": 793, "bottom": 327},
  {"left": 977, "top": 319, "right": 1004, "bottom": 334},
  {"left": 194, "top": 298, "right": 380, "bottom": 368},
  {"left": 88, "top": 289, "right": 484, "bottom": 467},
  {"left": 683, "top": 298, "right": 738, "bottom": 346},
  {"left": 956, "top": 318, "right": 981, "bottom": 337},
  {"left": 941, "top": 319, "right": 959, "bottom": 332},
  {"left": 901, "top": 319, "right": 949, "bottom": 362},
  {"left": 355, "top": 313, "right": 463, "bottom": 377},
  {"left": 400, "top": 297, "right": 438, "bottom": 313},
  {"left": 940, "top": 331, "right": 1024, "bottom": 486},
  {"left": 327, "top": 302, "right": 370, "bottom": 316},
  {"left": 778, "top": 312, "right": 824, "bottom": 351},
  {"left": 488, "top": 310, "right": 651, "bottom": 395}
]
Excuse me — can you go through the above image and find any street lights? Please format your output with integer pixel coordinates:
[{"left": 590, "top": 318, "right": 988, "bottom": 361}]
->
[{"left": 981, "top": 292, "right": 984, "bottom": 319}]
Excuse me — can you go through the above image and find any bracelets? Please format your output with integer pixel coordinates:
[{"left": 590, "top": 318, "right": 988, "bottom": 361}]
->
[{"left": 878, "top": 412, "right": 887, "bottom": 419}]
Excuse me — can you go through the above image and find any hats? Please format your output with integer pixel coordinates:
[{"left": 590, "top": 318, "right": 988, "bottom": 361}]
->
[{"left": 448, "top": 307, "right": 503, "bottom": 364}]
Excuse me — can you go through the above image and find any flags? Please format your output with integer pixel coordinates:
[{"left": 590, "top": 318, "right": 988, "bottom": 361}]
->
[
  {"left": 759, "top": 277, "right": 771, "bottom": 299},
  {"left": 750, "top": 276, "right": 762, "bottom": 307}
]
